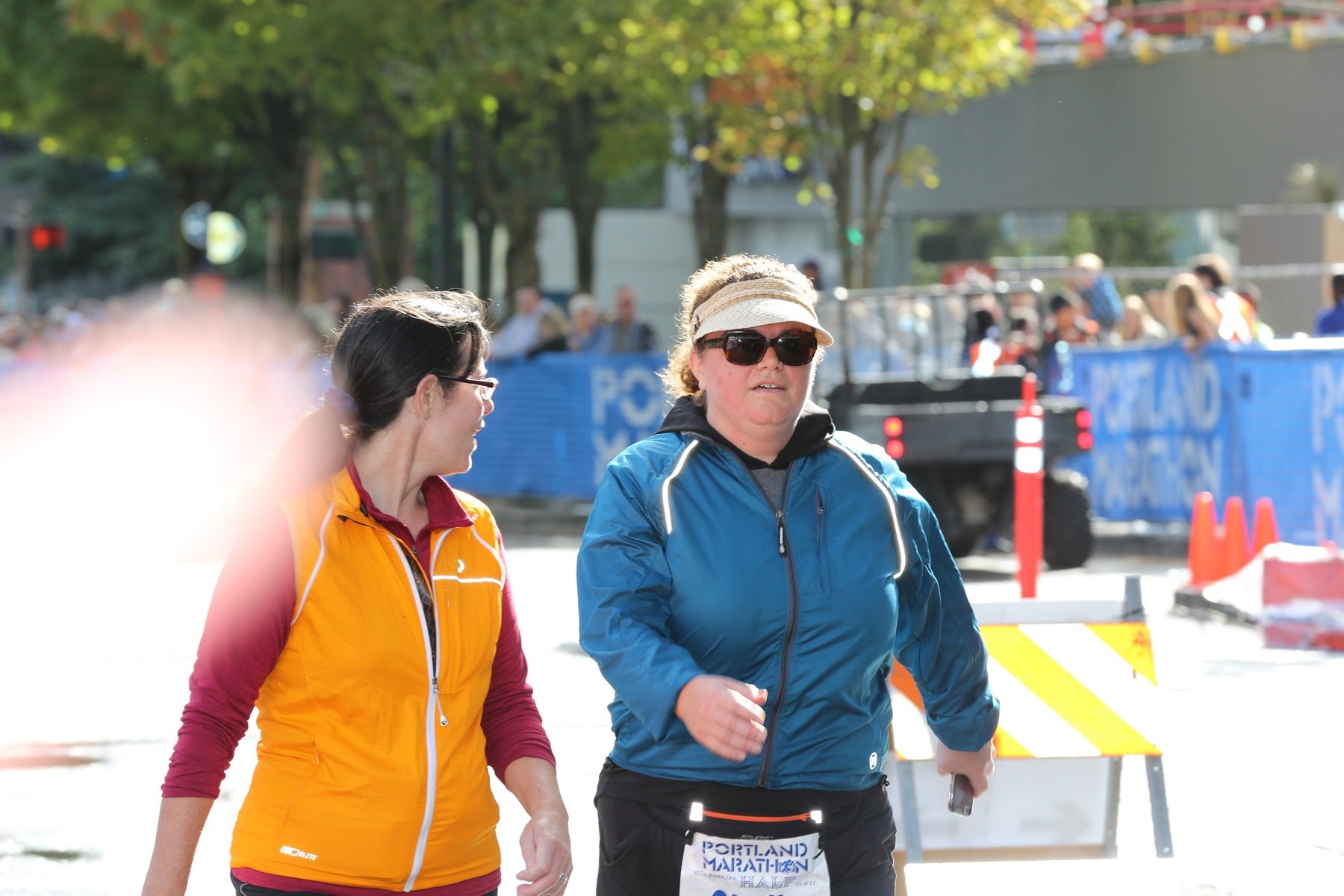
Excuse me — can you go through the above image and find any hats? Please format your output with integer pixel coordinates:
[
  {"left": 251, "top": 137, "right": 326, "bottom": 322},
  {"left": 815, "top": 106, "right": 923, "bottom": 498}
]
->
[{"left": 691, "top": 278, "right": 836, "bottom": 348}]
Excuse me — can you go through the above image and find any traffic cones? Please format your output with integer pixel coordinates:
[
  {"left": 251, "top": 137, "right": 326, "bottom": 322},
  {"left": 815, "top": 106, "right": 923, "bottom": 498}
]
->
[{"left": 1173, "top": 490, "right": 1280, "bottom": 625}]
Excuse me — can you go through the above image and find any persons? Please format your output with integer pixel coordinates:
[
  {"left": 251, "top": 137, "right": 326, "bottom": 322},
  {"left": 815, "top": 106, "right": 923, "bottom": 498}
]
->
[
  {"left": 941, "top": 250, "right": 1344, "bottom": 396},
  {"left": 573, "top": 253, "right": 1000, "bottom": 896},
  {"left": 479, "top": 280, "right": 658, "bottom": 369},
  {"left": 142, "top": 292, "right": 573, "bottom": 896}
]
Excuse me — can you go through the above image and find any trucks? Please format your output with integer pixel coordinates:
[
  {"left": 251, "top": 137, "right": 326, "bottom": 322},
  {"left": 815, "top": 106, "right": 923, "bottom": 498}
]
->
[{"left": 806, "top": 258, "right": 1097, "bottom": 572}]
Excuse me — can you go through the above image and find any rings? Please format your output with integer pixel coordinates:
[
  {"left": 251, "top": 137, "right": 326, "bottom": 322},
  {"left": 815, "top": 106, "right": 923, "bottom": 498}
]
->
[{"left": 559, "top": 872, "right": 568, "bottom": 884}]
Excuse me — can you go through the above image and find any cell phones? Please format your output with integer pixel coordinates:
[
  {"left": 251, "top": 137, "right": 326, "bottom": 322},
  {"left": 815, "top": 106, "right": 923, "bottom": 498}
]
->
[{"left": 947, "top": 773, "right": 973, "bottom": 816}]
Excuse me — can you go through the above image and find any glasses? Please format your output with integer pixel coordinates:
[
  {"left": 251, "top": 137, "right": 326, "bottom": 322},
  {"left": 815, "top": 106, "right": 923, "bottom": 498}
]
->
[
  {"left": 436, "top": 376, "right": 499, "bottom": 400},
  {"left": 700, "top": 329, "right": 818, "bottom": 367}
]
